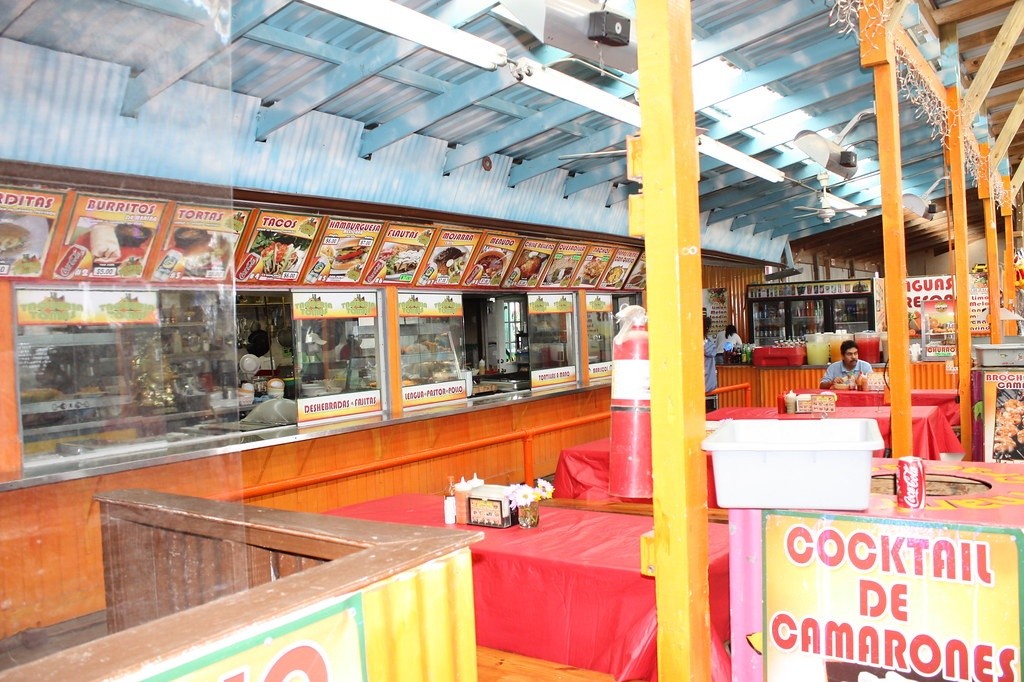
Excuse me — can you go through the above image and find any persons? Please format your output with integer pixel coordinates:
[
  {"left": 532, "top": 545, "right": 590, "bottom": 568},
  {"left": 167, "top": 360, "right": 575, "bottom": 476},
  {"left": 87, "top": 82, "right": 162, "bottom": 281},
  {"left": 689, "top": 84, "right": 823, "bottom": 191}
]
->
[
  {"left": 820, "top": 339, "right": 873, "bottom": 390},
  {"left": 716, "top": 324, "right": 743, "bottom": 365},
  {"left": 703, "top": 317, "right": 717, "bottom": 412}
]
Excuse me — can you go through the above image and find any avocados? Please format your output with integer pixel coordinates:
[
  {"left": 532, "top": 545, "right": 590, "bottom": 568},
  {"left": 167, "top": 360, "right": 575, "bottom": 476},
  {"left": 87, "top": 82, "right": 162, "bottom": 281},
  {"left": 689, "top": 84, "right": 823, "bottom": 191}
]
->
[{"left": 447, "top": 260, "right": 465, "bottom": 275}]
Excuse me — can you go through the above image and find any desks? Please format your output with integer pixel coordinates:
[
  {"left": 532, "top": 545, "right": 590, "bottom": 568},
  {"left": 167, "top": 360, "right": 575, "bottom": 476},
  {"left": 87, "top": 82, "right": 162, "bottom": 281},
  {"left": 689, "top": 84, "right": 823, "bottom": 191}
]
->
[
  {"left": 778, "top": 388, "right": 961, "bottom": 425},
  {"left": 705, "top": 406, "right": 967, "bottom": 461},
  {"left": 553, "top": 439, "right": 717, "bottom": 509},
  {"left": 318, "top": 491, "right": 731, "bottom": 682}
]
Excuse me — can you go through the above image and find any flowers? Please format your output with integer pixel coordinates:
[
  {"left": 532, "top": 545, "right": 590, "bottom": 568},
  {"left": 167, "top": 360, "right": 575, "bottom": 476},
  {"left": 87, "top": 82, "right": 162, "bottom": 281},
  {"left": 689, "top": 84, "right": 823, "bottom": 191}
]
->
[{"left": 503, "top": 479, "right": 555, "bottom": 526}]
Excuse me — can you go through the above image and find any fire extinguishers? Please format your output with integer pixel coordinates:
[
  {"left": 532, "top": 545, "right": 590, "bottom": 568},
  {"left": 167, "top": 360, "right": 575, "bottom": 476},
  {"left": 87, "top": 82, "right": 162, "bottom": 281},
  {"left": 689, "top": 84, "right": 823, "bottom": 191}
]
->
[{"left": 609, "top": 304, "right": 653, "bottom": 499}]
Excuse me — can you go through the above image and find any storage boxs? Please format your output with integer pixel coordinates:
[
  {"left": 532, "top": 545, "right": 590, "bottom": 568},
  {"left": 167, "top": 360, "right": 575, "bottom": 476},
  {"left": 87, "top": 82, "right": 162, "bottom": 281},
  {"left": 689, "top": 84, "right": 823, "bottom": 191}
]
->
[
  {"left": 699, "top": 420, "right": 884, "bottom": 511},
  {"left": 975, "top": 344, "right": 1024, "bottom": 366},
  {"left": 751, "top": 330, "right": 889, "bottom": 365}
]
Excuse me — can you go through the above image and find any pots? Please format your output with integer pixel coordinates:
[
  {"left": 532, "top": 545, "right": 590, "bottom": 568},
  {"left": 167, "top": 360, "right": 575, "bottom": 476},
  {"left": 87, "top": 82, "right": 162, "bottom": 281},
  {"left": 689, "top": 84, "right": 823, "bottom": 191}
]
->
[{"left": 250, "top": 375, "right": 285, "bottom": 400}]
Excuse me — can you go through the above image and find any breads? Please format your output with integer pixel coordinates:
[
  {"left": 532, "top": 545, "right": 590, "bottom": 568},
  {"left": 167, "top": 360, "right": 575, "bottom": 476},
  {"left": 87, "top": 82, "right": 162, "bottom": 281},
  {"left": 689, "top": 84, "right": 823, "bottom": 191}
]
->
[{"left": 401, "top": 340, "right": 441, "bottom": 354}]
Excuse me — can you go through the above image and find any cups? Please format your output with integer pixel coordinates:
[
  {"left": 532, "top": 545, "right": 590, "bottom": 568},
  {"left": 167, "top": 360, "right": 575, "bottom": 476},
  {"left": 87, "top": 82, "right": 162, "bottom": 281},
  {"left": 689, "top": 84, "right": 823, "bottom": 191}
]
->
[{"left": 777, "top": 395, "right": 787, "bottom": 414}]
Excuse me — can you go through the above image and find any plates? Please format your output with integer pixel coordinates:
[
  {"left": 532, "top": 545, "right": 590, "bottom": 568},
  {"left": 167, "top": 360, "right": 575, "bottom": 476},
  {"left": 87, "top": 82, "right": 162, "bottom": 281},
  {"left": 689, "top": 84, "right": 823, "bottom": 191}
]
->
[
  {"left": 628, "top": 274, "right": 645, "bottom": 285},
  {"left": 74, "top": 392, "right": 110, "bottom": 398},
  {"left": 602, "top": 269, "right": 624, "bottom": 285},
  {"left": 330, "top": 238, "right": 470, "bottom": 279},
  {"left": 239, "top": 353, "right": 260, "bottom": 374}
]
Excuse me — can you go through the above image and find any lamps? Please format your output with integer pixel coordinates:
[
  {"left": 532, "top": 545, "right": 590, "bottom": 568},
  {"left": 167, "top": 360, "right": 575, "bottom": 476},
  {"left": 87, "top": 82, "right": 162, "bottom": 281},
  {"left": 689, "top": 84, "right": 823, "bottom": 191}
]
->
[
  {"left": 901, "top": 175, "right": 950, "bottom": 220},
  {"left": 791, "top": 99, "right": 876, "bottom": 180},
  {"left": 540, "top": 1, "right": 641, "bottom": 77}
]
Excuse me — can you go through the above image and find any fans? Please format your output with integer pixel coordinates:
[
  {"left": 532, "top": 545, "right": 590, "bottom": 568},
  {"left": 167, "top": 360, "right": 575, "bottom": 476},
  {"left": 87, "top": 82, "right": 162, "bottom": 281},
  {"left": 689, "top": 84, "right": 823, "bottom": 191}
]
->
[
  {"left": 791, "top": 173, "right": 881, "bottom": 229},
  {"left": 558, "top": 89, "right": 710, "bottom": 160}
]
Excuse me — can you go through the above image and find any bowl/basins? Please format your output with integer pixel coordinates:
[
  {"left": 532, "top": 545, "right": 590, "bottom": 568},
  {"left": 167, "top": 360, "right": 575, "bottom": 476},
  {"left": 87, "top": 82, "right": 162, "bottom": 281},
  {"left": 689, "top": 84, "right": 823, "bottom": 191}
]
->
[
  {"left": 174, "top": 228, "right": 208, "bottom": 248},
  {"left": 477, "top": 250, "right": 507, "bottom": 275},
  {"left": 114, "top": 224, "right": 152, "bottom": 248},
  {"left": 469, "top": 369, "right": 480, "bottom": 375},
  {"left": 833, "top": 383, "right": 850, "bottom": 390}
]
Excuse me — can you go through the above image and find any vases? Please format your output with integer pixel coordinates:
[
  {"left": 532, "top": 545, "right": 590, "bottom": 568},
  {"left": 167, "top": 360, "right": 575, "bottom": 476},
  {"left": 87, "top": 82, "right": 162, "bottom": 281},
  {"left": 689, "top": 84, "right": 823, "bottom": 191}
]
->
[{"left": 518, "top": 503, "right": 539, "bottom": 528}]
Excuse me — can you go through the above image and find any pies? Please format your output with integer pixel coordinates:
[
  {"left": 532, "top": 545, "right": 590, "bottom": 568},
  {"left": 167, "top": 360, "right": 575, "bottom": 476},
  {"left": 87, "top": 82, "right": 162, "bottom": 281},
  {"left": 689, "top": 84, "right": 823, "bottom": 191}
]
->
[
  {"left": 89, "top": 223, "right": 121, "bottom": 263},
  {"left": 260, "top": 242, "right": 297, "bottom": 274}
]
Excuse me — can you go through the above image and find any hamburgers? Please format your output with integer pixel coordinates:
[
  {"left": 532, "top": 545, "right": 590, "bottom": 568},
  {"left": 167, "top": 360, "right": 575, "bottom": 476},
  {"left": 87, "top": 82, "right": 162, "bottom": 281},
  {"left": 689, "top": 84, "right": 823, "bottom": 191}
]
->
[
  {"left": 0, "top": 222, "right": 31, "bottom": 259},
  {"left": 332, "top": 238, "right": 365, "bottom": 270}
]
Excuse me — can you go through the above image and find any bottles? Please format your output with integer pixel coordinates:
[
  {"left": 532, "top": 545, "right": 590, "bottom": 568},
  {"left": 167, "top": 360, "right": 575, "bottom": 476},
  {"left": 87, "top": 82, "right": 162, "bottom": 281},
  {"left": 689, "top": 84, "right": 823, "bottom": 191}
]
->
[
  {"left": 170, "top": 304, "right": 210, "bottom": 354},
  {"left": 755, "top": 300, "right": 868, "bottom": 348},
  {"left": 479, "top": 358, "right": 485, "bottom": 375},
  {"left": 467, "top": 473, "right": 484, "bottom": 489},
  {"left": 785, "top": 390, "right": 796, "bottom": 414},
  {"left": 454, "top": 477, "right": 469, "bottom": 525},
  {"left": 850, "top": 371, "right": 863, "bottom": 391},
  {"left": 443, "top": 476, "right": 457, "bottom": 525},
  {"left": 724, "top": 343, "right": 752, "bottom": 366}
]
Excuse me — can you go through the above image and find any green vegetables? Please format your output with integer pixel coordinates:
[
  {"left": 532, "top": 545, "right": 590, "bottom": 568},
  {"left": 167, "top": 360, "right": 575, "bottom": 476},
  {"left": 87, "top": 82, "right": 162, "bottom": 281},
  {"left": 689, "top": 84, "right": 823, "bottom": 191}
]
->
[{"left": 249, "top": 230, "right": 314, "bottom": 250}]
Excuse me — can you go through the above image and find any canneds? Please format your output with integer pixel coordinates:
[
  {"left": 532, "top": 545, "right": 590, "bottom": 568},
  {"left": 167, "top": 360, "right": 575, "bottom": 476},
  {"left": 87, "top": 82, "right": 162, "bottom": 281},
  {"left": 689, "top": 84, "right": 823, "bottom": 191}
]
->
[
  {"left": 896, "top": 456, "right": 925, "bottom": 510},
  {"left": 236, "top": 252, "right": 260, "bottom": 281},
  {"left": 55, "top": 243, "right": 88, "bottom": 277},
  {"left": 365, "top": 260, "right": 386, "bottom": 283},
  {"left": 806, "top": 300, "right": 819, "bottom": 316},
  {"left": 306, "top": 258, "right": 327, "bottom": 285},
  {"left": 504, "top": 267, "right": 520, "bottom": 287},
  {"left": 153, "top": 249, "right": 183, "bottom": 280},
  {"left": 756, "top": 330, "right": 777, "bottom": 337},
  {"left": 465, "top": 265, "right": 481, "bottom": 285},
  {"left": 418, "top": 262, "right": 438, "bottom": 285}
]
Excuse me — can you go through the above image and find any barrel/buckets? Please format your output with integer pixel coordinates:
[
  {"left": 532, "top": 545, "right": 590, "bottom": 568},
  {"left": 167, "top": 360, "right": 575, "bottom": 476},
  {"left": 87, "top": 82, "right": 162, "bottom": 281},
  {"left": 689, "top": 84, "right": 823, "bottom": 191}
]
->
[{"left": 802, "top": 329, "right": 889, "bottom": 365}]
